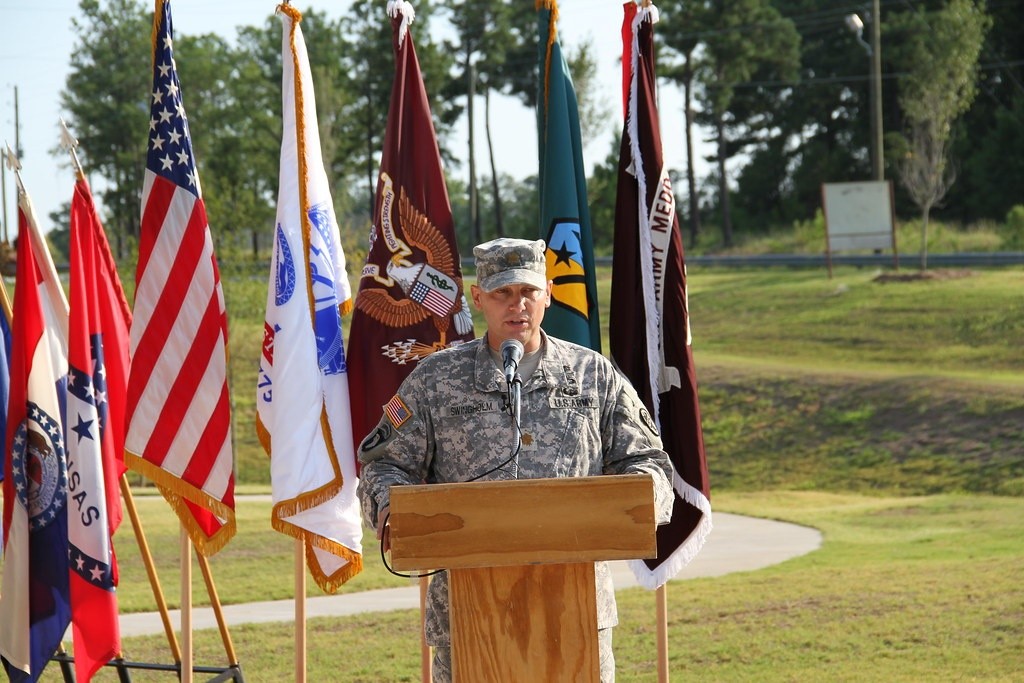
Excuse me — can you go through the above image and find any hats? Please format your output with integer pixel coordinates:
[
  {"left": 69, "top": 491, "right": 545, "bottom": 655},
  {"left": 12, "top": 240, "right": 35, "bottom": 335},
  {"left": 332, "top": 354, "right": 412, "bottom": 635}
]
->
[{"left": 473, "top": 237, "right": 548, "bottom": 293}]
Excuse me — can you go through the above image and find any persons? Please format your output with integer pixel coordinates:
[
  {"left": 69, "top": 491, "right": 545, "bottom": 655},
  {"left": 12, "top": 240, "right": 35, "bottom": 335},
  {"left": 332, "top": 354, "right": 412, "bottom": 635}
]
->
[{"left": 355, "top": 238, "right": 677, "bottom": 683}]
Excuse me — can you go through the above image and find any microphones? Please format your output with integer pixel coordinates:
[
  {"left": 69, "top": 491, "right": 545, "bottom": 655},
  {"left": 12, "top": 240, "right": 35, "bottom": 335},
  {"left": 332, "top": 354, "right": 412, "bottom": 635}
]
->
[{"left": 500, "top": 338, "right": 525, "bottom": 385}]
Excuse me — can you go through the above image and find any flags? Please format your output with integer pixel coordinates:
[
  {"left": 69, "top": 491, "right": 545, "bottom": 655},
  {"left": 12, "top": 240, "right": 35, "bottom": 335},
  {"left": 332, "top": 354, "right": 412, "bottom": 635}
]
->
[
  {"left": 256, "top": 0, "right": 367, "bottom": 593},
  {"left": 608, "top": 0, "right": 713, "bottom": 593},
  {"left": 0, "top": 182, "right": 70, "bottom": 683},
  {"left": 345, "top": 0, "right": 477, "bottom": 479},
  {"left": 539, "top": 1, "right": 603, "bottom": 353},
  {"left": 61, "top": 173, "right": 133, "bottom": 683},
  {"left": 121, "top": 0, "right": 237, "bottom": 555}
]
